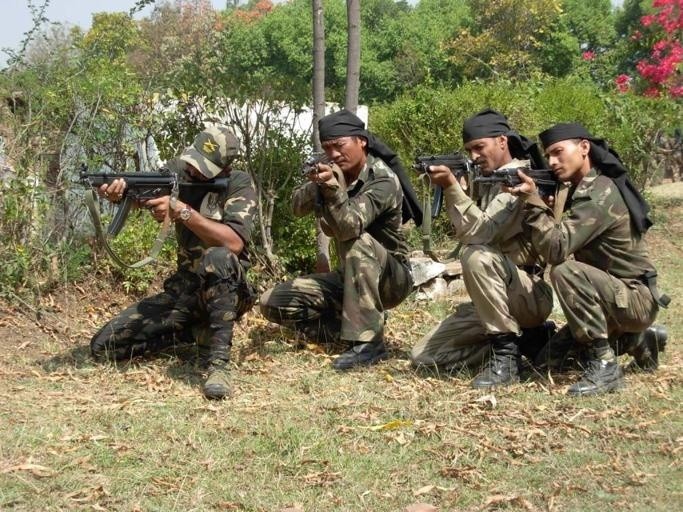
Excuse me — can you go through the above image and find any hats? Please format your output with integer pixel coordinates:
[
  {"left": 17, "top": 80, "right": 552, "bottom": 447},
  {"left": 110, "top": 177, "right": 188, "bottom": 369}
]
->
[{"left": 180, "top": 127, "right": 240, "bottom": 178}]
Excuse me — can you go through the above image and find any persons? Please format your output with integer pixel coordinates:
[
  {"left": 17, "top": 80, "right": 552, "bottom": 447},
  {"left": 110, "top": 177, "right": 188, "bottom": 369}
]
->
[
  {"left": 407, "top": 110, "right": 557, "bottom": 392},
  {"left": 89, "top": 126, "right": 258, "bottom": 397},
  {"left": 258, "top": 111, "right": 425, "bottom": 371},
  {"left": 498, "top": 121, "right": 668, "bottom": 396}
]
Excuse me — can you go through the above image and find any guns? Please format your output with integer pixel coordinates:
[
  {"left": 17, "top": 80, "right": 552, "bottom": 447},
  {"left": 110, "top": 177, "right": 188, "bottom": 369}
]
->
[
  {"left": 71, "top": 160, "right": 230, "bottom": 242},
  {"left": 300, "top": 154, "right": 329, "bottom": 218},
  {"left": 414, "top": 151, "right": 484, "bottom": 220},
  {"left": 471, "top": 167, "right": 557, "bottom": 191}
]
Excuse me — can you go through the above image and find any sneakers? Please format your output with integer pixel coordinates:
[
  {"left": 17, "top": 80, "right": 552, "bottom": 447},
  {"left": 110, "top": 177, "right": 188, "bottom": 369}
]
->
[{"left": 204, "top": 372, "right": 231, "bottom": 396}]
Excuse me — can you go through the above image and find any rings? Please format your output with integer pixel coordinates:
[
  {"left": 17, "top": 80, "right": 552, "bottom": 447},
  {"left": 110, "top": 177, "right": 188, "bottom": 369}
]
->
[
  {"left": 117, "top": 194, "right": 122, "bottom": 199},
  {"left": 103, "top": 192, "right": 108, "bottom": 199}
]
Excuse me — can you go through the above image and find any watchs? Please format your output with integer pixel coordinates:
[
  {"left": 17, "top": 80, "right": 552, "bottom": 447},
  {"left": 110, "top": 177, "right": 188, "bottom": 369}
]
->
[{"left": 178, "top": 202, "right": 191, "bottom": 224}]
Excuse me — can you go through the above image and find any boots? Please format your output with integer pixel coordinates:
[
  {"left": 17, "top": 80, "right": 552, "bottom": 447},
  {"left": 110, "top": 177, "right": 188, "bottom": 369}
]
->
[
  {"left": 568, "top": 348, "right": 624, "bottom": 397},
  {"left": 521, "top": 322, "right": 557, "bottom": 361},
  {"left": 626, "top": 325, "right": 667, "bottom": 374},
  {"left": 471, "top": 333, "right": 521, "bottom": 388}
]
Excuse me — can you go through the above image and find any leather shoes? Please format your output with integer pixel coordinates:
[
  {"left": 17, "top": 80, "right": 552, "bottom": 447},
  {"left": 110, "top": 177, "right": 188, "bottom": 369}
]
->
[{"left": 333, "top": 340, "right": 387, "bottom": 369}]
